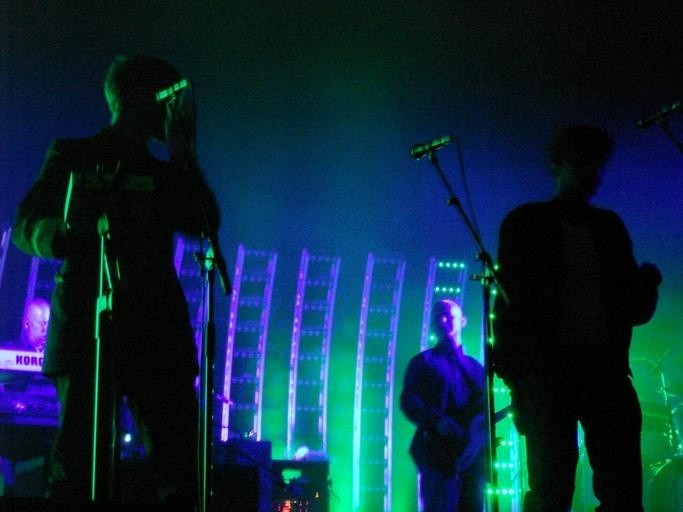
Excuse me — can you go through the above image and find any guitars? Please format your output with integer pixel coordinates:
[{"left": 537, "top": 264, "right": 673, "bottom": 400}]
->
[{"left": 427, "top": 403, "right": 514, "bottom": 474}]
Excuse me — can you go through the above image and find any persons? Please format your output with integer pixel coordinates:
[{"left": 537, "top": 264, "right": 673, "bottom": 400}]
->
[
  {"left": 10, "top": 54, "right": 224, "bottom": 512},
  {"left": 398, "top": 299, "right": 498, "bottom": 512},
  {"left": 0, "top": 297, "right": 52, "bottom": 490},
  {"left": 492, "top": 123, "right": 663, "bottom": 512}
]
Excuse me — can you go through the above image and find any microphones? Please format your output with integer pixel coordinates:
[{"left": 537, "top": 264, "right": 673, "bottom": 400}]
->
[
  {"left": 634, "top": 99, "right": 683, "bottom": 130},
  {"left": 153, "top": 76, "right": 192, "bottom": 105},
  {"left": 409, "top": 134, "right": 456, "bottom": 161}
]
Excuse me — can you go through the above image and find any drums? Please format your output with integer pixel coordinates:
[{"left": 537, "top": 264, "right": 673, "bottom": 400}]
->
[{"left": 642, "top": 453, "right": 683, "bottom": 512}]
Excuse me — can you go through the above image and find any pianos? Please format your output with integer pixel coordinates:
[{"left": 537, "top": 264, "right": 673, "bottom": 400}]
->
[{"left": 0, "top": 346, "right": 44, "bottom": 373}]
